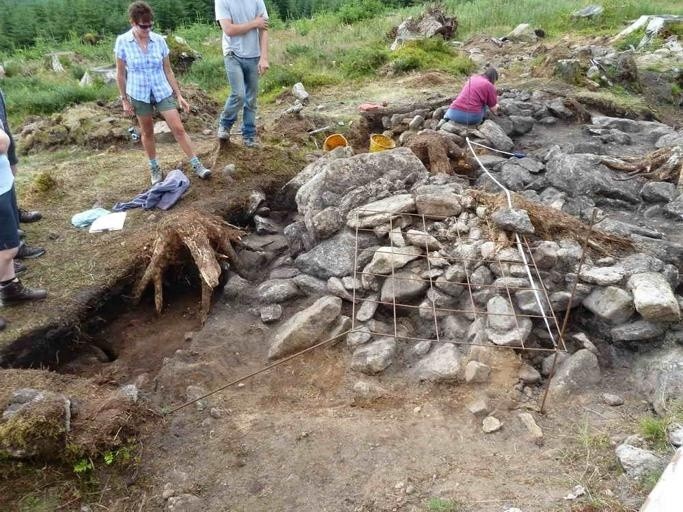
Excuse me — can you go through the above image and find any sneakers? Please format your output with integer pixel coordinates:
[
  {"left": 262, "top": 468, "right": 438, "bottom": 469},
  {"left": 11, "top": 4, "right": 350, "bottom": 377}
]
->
[
  {"left": 193, "top": 162, "right": 212, "bottom": 180},
  {"left": 14, "top": 244, "right": 45, "bottom": 274},
  {"left": 217, "top": 126, "right": 230, "bottom": 140},
  {"left": 20, "top": 210, "right": 43, "bottom": 223},
  {"left": 244, "top": 139, "right": 260, "bottom": 148},
  {"left": 1, "top": 280, "right": 48, "bottom": 304},
  {"left": 150, "top": 166, "right": 163, "bottom": 186}
]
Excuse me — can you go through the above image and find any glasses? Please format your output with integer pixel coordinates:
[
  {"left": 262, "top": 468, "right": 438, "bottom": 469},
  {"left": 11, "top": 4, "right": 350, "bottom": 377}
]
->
[{"left": 137, "top": 23, "right": 152, "bottom": 29}]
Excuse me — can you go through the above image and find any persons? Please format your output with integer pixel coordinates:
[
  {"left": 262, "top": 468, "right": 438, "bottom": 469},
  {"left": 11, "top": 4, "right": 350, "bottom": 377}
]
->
[
  {"left": 113, "top": 2, "right": 212, "bottom": 185},
  {"left": 0, "top": 65, "right": 48, "bottom": 330},
  {"left": 435, "top": 67, "right": 501, "bottom": 130},
  {"left": 215, "top": 1, "right": 270, "bottom": 149}
]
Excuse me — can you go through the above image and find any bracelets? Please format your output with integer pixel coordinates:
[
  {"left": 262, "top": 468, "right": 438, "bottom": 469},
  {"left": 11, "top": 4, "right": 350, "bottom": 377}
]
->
[{"left": 120, "top": 96, "right": 127, "bottom": 101}]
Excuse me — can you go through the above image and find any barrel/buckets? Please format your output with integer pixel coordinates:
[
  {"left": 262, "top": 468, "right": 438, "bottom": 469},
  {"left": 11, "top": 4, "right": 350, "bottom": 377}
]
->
[
  {"left": 368, "top": 133, "right": 395, "bottom": 151},
  {"left": 322, "top": 134, "right": 347, "bottom": 151}
]
